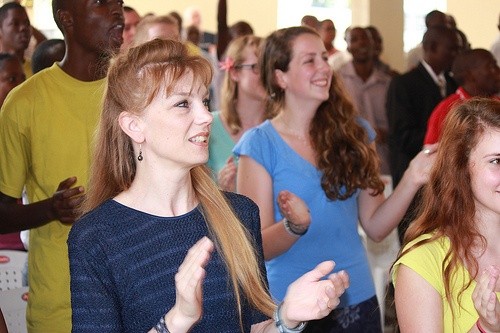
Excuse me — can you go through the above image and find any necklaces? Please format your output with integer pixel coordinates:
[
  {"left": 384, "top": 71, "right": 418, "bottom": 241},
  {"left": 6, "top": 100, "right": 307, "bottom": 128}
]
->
[{"left": 279, "top": 115, "right": 314, "bottom": 149}]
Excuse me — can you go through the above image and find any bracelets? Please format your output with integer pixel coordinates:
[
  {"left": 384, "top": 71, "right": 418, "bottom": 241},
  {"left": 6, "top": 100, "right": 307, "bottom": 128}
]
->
[
  {"left": 282, "top": 217, "right": 309, "bottom": 238},
  {"left": 273, "top": 300, "right": 307, "bottom": 333},
  {"left": 154, "top": 312, "right": 171, "bottom": 333}
]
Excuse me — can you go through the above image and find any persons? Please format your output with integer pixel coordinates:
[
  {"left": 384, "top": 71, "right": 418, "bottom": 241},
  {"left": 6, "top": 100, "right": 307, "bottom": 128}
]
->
[
  {"left": 334, "top": 25, "right": 393, "bottom": 174},
  {"left": 0, "top": 0, "right": 500, "bottom": 333},
  {"left": 230, "top": 26, "right": 440, "bottom": 333},
  {"left": 204, "top": 35, "right": 283, "bottom": 194}
]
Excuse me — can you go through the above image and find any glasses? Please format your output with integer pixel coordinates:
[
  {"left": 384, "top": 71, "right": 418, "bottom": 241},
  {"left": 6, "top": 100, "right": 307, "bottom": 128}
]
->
[{"left": 235, "top": 62, "right": 259, "bottom": 75}]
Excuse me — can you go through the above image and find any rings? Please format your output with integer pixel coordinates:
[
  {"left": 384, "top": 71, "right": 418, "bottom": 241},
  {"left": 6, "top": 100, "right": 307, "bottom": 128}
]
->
[
  {"left": 327, "top": 302, "right": 332, "bottom": 313},
  {"left": 423, "top": 147, "right": 431, "bottom": 157}
]
